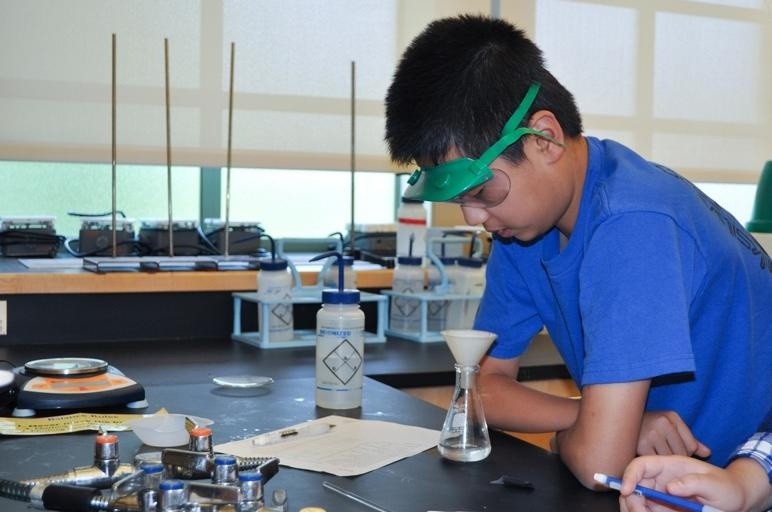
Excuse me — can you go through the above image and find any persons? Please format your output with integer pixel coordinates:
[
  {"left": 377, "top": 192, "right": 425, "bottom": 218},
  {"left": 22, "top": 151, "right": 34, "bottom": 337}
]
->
[
  {"left": 381, "top": 11, "right": 772, "bottom": 493},
  {"left": 616, "top": 411, "right": 772, "bottom": 512}
]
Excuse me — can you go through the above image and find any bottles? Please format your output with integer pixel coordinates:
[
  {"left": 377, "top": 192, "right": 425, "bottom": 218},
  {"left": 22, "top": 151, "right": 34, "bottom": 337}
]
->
[
  {"left": 393, "top": 197, "right": 484, "bottom": 336},
  {"left": 438, "top": 329, "right": 497, "bottom": 463},
  {"left": 257, "top": 255, "right": 365, "bottom": 409},
  {"left": 97, "top": 422, "right": 264, "bottom": 511}
]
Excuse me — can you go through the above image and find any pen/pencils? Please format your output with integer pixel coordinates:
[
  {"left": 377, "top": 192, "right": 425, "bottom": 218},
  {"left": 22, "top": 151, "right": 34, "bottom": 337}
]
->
[{"left": 593, "top": 473, "right": 724, "bottom": 512}]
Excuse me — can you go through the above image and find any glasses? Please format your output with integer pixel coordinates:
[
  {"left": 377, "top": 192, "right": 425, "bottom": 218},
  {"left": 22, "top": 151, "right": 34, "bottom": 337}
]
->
[{"left": 403, "top": 78, "right": 575, "bottom": 209}]
